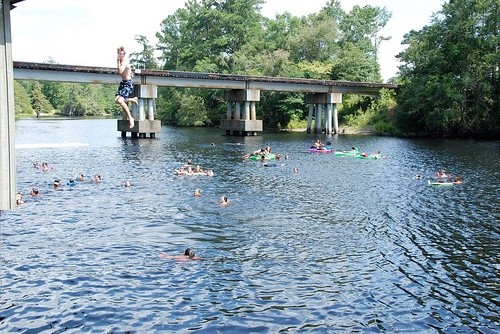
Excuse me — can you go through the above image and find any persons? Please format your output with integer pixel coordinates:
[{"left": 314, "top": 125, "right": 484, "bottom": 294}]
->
[
  {"left": 42, "top": 162, "right": 48, "bottom": 169},
  {"left": 185, "top": 248, "right": 195, "bottom": 258},
  {"left": 54, "top": 174, "right": 101, "bottom": 188},
  {"left": 115, "top": 47, "right": 138, "bottom": 128},
  {"left": 242, "top": 146, "right": 280, "bottom": 161},
  {"left": 293, "top": 168, "right": 298, "bottom": 172},
  {"left": 285, "top": 155, "right": 288, "bottom": 159},
  {"left": 343, "top": 146, "right": 355, "bottom": 154},
  {"left": 33, "top": 162, "right": 39, "bottom": 169},
  {"left": 125, "top": 180, "right": 130, "bottom": 186},
  {"left": 361, "top": 150, "right": 387, "bottom": 159},
  {"left": 194, "top": 189, "right": 200, "bottom": 196},
  {"left": 31, "top": 188, "right": 38, "bottom": 196},
  {"left": 180, "top": 162, "right": 212, "bottom": 176},
  {"left": 315, "top": 139, "right": 332, "bottom": 151},
  {"left": 220, "top": 196, "right": 229, "bottom": 206},
  {"left": 436, "top": 169, "right": 461, "bottom": 183}
]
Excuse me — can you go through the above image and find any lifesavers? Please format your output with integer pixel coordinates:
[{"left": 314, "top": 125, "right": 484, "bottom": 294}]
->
[{"left": 186, "top": 172, "right": 214, "bottom": 175}]
